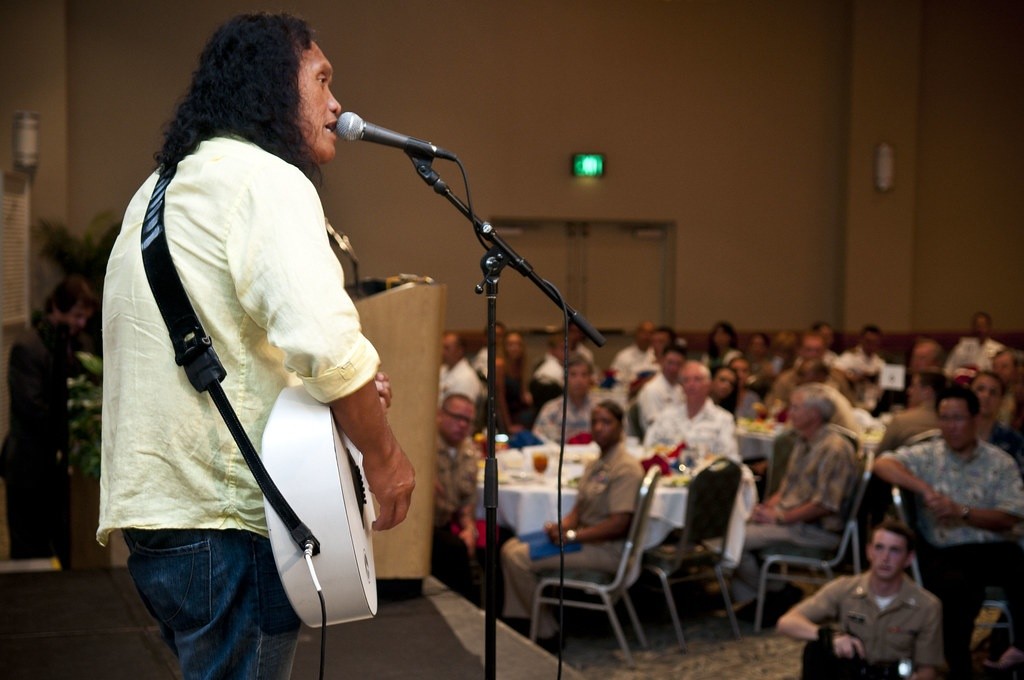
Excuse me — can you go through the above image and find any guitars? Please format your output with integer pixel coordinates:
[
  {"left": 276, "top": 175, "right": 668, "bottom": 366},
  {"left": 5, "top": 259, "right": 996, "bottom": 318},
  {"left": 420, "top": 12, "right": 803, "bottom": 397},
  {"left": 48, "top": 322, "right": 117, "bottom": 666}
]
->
[{"left": 257, "top": 381, "right": 380, "bottom": 632}]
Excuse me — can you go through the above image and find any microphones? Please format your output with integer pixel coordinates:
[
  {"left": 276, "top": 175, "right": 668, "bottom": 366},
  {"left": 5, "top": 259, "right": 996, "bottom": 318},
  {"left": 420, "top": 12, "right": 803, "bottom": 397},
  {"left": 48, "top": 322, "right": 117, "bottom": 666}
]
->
[
  {"left": 337, "top": 112, "right": 456, "bottom": 161},
  {"left": 324, "top": 218, "right": 348, "bottom": 253}
]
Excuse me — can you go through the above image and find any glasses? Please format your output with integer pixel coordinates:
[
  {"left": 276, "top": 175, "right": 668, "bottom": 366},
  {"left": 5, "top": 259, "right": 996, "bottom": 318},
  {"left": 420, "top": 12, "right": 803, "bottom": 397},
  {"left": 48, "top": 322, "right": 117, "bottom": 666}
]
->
[{"left": 444, "top": 408, "right": 472, "bottom": 423}]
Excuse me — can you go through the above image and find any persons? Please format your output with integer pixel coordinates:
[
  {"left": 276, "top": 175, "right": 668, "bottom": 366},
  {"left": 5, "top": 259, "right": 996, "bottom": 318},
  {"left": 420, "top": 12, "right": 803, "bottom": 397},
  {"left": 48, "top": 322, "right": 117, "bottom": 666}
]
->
[
  {"left": 0, "top": 277, "right": 101, "bottom": 576},
  {"left": 429, "top": 311, "right": 1024, "bottom": 680},
  {"left": 777, "top": 520, "right": 944, "bottom": 680},
  {"left": 96, "top": 11, "right": 416, "bottom": 680}
]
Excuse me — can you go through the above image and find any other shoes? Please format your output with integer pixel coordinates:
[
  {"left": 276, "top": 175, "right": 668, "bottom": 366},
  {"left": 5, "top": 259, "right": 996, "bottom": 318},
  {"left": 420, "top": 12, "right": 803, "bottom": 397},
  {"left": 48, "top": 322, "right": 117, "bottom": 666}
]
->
[
  {"left": 535, "top": 630, "right": 567, "bottom": 655},
  {"left": 734, "top": 583, "right": 805, "bottom": 628}
]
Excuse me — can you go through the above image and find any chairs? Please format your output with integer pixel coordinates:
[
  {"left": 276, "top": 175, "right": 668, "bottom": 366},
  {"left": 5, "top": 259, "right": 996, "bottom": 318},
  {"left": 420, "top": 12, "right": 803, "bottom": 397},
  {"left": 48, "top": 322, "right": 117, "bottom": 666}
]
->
[
  {"left": 638, "top": 455, "right": 743, "bottom": 653},
  {"left": 530, "top": 463, "right": 663, "bottom": 670},
  {"left": 893, "top": 486, "right": 1018, "bottom": 649},
  {"left": 753, "top": 447, "right": 876, "bottom": 637}
]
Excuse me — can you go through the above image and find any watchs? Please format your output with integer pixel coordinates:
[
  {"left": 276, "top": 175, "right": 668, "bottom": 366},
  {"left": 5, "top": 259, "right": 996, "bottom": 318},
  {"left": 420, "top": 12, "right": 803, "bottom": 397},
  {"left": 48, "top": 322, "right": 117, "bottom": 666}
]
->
[{"left": 566, "top": 530, "right": 577, "bottom": 541}]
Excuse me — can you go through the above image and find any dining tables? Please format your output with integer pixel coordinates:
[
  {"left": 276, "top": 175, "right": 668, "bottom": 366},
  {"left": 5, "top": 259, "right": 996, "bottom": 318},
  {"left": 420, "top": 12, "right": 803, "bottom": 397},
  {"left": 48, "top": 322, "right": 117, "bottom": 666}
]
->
[
  {"left": 733, "top": 422, "right": 787, "bottom": 461},
  {"left": 475, "top": 441, "right": 689, "bottom": 553}
]
